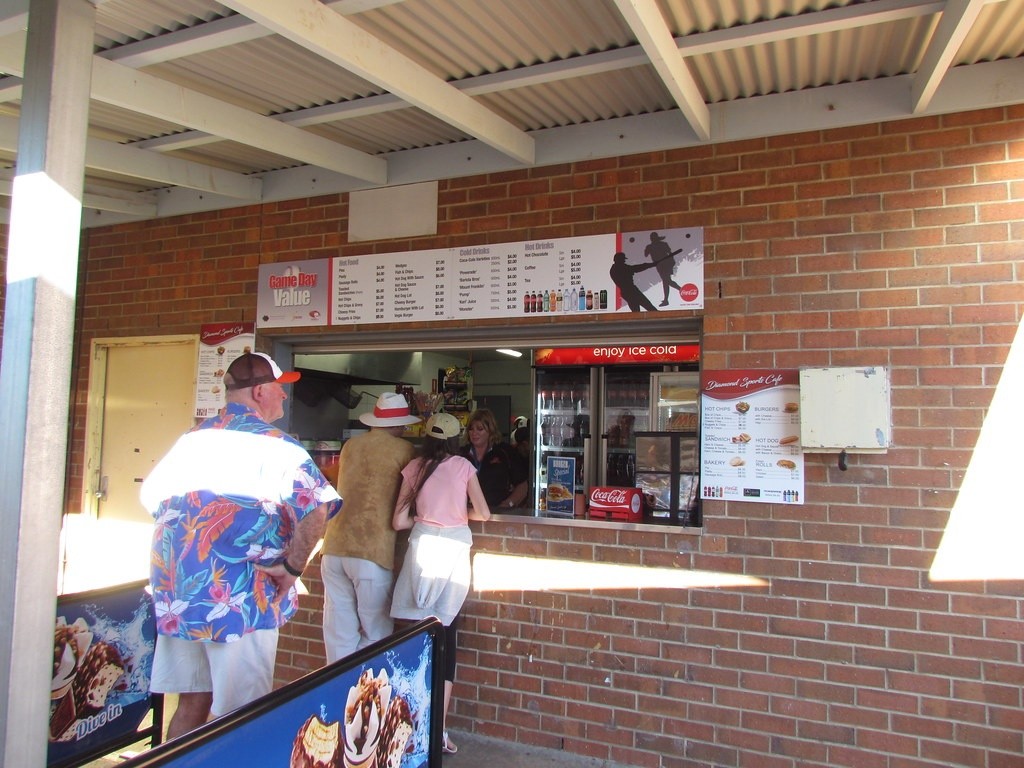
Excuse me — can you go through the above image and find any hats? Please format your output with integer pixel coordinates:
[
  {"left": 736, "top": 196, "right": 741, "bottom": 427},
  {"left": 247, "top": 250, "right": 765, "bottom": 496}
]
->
[
  {"left": 426, "top": 413, "right": 460, "bottom": 439},
  {"left": 225, "top": 352, "right": 301, "bottom": 390},
  {"left": 359, "top": 392, "right": 422, "bottom": 427}
]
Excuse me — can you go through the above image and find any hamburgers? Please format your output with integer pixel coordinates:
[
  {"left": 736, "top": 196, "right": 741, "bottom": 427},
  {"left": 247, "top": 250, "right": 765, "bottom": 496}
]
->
[
  {"left": 784, "top": 403, "right": 798, "bottom": 412},
  {"left": 548, "top": 483, "right": 563, "bottom": 501}
]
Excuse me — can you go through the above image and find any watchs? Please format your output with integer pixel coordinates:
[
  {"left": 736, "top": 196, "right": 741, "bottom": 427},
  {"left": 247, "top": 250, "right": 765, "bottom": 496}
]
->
[{"left": 506, "top": 496, "right": 515, "bottom": 507}]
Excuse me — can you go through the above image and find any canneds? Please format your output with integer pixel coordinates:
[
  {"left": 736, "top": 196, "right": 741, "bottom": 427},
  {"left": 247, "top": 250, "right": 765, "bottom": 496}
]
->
[{"left": 600, "top": 290, "right": 607, "bottom": 309}]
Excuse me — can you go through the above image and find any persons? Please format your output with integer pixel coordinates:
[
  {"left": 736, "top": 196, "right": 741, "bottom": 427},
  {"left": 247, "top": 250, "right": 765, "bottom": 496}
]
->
[
  {"left": 319, "top": 391, "right": 422, "bottom": 665},
  {"left": 392, "top": 413, "right": 490, "bottom": 753},
  {"left": 559, "top": 410, "right": 638, "bottom": 486},
  {"left": 137, "top": 351, "right": 344, "bottom": 745},
  {"left": 460, "top": 409, "right": 531, "bottom": 508}
]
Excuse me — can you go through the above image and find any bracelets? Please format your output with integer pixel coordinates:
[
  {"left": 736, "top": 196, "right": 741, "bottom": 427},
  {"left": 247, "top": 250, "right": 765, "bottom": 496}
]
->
[{"left": 283, "top": 558, "right": 303, "bottom": 576}]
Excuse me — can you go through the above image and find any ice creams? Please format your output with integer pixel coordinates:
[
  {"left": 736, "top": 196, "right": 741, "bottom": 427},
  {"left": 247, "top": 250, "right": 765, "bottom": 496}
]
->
[
  {"left": 289, "top": 668, "right": 393, "bottom": 768},
  {"left": 52, "top": 616, "right": 94, "bottom": 700}
]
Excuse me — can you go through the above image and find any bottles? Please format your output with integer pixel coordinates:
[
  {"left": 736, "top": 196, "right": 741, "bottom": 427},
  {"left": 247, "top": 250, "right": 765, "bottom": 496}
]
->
[
  {"left": 579, "top": 285, "right": 585, "bottom": 310},
  {"left": 556, "top": 289, "right": 563, "bottom": 311},
  {"left": 537, "top": 291, "right": 543, "bottom": 312},
  {"left": 600, "top": 290, "right": 607, "bottom": 309},
  {"left": 549, "top": 290, "right": 556, "bottom": 312},
  {"left": 539, "top": 414, "right": 590, "bottom": 446},
  {"left": 586, "top": 290, "right": 593, "bottom": 310},
  {"left": 563, "top": 289, "right": 570, "bottom": 311},
  {"left": 704, "top": 486, "right": 723, "bottom": 497},
  {"left": 783, "top": 490, "right": 798, "bottom": 502},
  {"left": 570, "top": 288, "right": 578, "bottom": 311},
  {"left": 524, "top": 291, "right": 530, "bottom": 312},
  {"left": 544, "top": 290, "right": 549, "bottom": 312},
  {"left": 531, "top": 291, "right": 536, "bottom": 312},
  {"left": 542, "top": 372, "right": 650, "bottom": 409},
  {"left": 302, "top": 439, "right": 340, "bottom": 490},
  {"left": 594, "top": 293, "right": 599, "bottom": 309},
  {"left": 539, "top": 450, "right": 585, "bottom": 516},
  {"left": 603, "top": 411, "right": 637, "bottom": 487}
]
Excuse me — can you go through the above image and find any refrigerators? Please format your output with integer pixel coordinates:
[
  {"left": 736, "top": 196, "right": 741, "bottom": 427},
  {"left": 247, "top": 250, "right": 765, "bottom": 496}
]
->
[{"left": 529, "top": 344, "right": 703, "bottom": 526}]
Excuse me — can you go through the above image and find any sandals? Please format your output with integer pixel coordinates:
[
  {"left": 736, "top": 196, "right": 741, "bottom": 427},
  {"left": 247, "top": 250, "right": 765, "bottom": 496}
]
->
[{"left": 442, "top": 732, "right": 457, "bottom": 753}]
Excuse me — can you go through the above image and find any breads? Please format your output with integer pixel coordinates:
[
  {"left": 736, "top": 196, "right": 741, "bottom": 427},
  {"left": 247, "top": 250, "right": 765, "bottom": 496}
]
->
[
  {"left": 730, "top": 457, "right": 742, "bottom": 466},
  {"left": 779, "top": 436, "right": 798, "bottom": 444}
]
